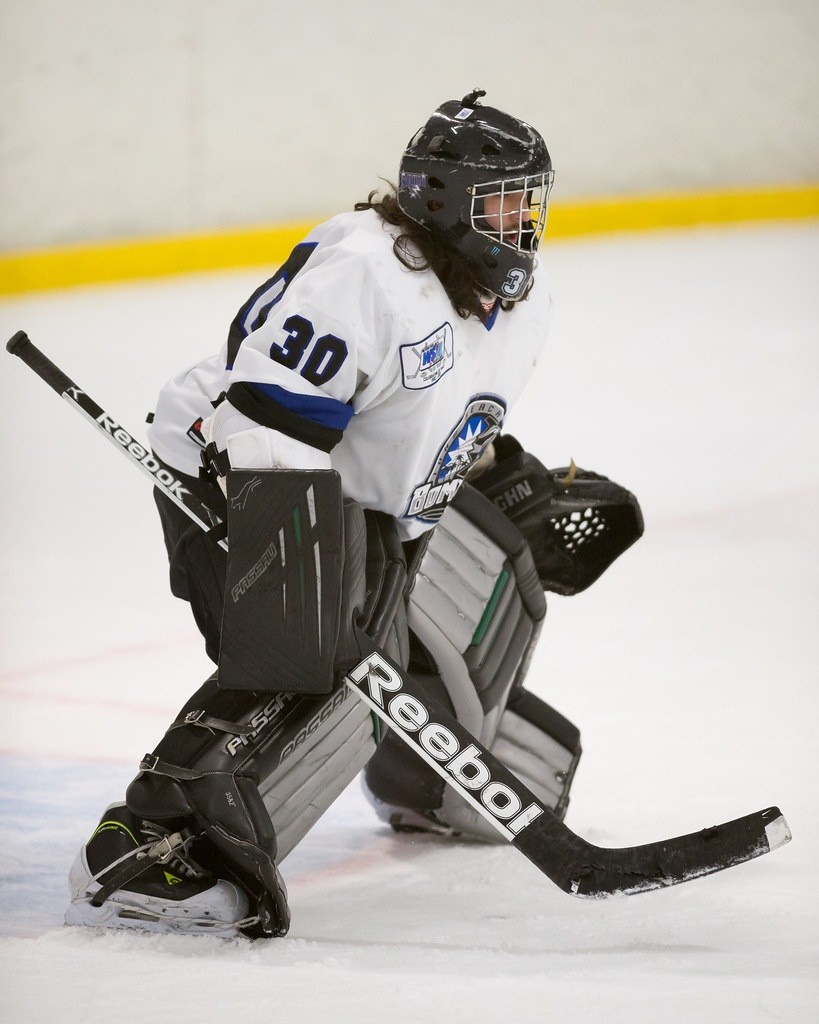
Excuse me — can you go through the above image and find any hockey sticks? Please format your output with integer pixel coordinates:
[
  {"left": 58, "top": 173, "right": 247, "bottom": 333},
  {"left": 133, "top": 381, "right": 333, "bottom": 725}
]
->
[{"left": 7, "top": 327, "right": 795, "bottom": 902}]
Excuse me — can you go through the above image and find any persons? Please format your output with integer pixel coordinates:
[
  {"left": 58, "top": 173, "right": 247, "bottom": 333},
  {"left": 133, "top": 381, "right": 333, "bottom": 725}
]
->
[{"left": 64, "top": 86, "right": 644, "bottom": 941}]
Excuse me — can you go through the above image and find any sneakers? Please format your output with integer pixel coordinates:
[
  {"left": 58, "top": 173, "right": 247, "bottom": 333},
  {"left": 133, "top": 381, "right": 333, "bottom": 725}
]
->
[
  {"left": 66, "top": 797, "right": 249, "bottom": 937},
  {"left": 361, "top": 729, "right": 515, "bottom": 844}
]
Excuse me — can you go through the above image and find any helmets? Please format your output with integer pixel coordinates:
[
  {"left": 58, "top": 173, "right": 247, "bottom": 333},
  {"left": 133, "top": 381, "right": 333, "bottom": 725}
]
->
[{"left": 397, "top": 90, "right": 554, "bottom": 301}]
